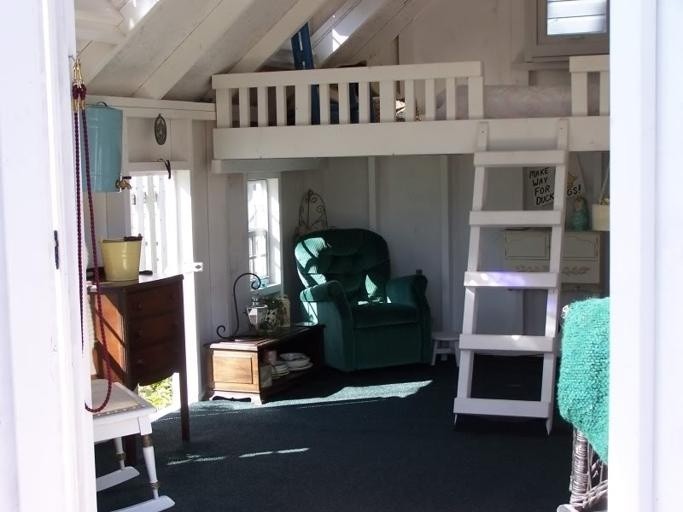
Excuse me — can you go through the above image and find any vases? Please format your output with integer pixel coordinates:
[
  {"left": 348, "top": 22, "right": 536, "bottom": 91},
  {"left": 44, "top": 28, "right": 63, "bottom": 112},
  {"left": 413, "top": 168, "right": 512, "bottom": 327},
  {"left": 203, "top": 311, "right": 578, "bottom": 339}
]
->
[{"left": 99, "top": 234, "right": 144, "bottom": 283}]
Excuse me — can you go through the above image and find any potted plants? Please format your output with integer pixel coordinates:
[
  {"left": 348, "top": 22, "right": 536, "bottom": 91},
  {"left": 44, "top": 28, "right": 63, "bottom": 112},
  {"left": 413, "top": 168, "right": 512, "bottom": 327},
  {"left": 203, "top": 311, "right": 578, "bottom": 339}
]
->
[{"left": 241, "top": 297, "right": 287, "bottom": 334}]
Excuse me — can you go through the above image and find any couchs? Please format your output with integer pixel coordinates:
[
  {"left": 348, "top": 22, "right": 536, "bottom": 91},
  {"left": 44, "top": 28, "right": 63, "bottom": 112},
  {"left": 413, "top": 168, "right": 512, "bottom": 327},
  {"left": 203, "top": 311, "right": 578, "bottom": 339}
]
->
[{"left": 292, "top": 227, "right": 430, "bottom": 372}]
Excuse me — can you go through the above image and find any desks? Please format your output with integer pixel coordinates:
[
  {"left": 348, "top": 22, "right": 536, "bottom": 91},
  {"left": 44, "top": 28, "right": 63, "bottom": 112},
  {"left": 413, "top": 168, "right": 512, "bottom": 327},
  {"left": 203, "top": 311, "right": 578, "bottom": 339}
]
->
[{"left": 90, "top": 377, "right": 175, "bottom": 512}]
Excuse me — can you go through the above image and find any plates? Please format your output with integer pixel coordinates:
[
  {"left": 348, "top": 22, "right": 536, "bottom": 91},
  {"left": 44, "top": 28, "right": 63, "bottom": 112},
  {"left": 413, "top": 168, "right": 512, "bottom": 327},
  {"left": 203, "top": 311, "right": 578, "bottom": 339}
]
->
[{"left": 259, "top": 350, "right": 313, "bottom": 389}]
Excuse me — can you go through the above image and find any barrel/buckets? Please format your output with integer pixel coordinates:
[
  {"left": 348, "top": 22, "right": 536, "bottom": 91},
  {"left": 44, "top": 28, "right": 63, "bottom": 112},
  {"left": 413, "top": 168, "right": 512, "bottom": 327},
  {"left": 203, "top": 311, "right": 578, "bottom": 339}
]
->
[{"left": 100, "top": 237, "right": 141, "bottom": 282}]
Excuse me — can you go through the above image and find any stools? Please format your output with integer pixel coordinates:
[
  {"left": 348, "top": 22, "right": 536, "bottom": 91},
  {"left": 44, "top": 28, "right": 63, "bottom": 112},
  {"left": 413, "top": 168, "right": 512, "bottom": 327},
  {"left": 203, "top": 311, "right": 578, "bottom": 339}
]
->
[{"left": 430, "top": 332, "right": 464, "bottom": 369}]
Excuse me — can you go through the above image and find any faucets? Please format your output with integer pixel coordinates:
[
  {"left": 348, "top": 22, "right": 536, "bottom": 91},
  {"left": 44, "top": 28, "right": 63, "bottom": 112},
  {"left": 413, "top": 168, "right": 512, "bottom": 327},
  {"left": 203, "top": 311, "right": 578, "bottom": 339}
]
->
[{"left": 116, "top": 175, "right": 133, "bottom": 190}]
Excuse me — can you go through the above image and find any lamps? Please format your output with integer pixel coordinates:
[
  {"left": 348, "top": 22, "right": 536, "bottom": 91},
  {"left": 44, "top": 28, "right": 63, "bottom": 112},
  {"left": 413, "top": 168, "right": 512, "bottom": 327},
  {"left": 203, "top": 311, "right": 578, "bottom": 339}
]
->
[{"left": 213, "top": 271, "right": 269, "bottom": 340}]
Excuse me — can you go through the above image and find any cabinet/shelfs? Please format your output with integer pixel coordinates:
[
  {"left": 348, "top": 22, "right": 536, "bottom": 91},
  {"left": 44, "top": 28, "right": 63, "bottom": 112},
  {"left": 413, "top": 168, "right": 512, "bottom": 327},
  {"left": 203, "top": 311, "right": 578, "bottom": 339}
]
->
[
  {"left": 496, "top": 224, "right": 603, "bottom": 295},
  {"left": 201, "top": 317, "right": 327, "bottom": 408},
  {"left": 87, "top": 269, "right": 190, "bottom": 469}
]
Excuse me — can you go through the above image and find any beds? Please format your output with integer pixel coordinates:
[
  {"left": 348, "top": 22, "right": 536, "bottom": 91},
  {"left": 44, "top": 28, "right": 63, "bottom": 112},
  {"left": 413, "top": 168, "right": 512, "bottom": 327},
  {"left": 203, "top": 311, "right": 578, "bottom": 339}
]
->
[{"left": 210, "top": 51, "right": 609, "bottom": 165}]
